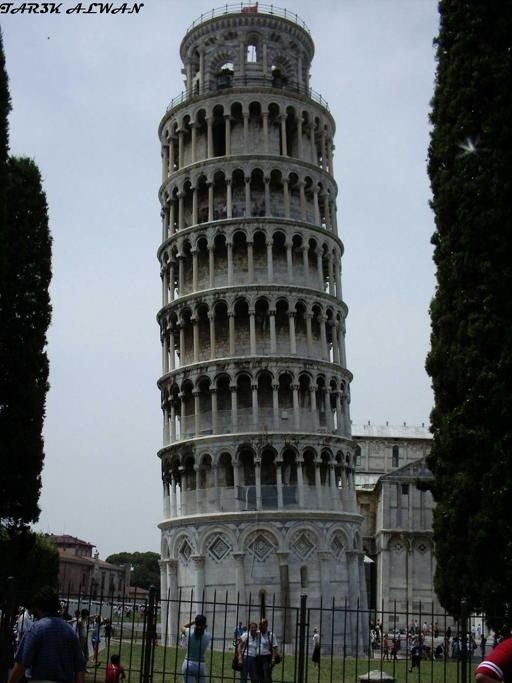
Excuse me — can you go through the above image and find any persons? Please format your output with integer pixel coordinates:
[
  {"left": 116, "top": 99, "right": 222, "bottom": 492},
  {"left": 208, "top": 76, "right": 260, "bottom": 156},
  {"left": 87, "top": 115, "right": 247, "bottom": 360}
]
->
[{"left": 0, "top": 592, "right": 510, "bottom": 683}]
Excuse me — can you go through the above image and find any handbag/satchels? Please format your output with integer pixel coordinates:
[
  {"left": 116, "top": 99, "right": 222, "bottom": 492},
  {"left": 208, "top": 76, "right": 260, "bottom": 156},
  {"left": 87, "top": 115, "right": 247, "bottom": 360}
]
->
[
  {"left": 268, "top": 653, "right": 282, "bottom": 664},
  {"left": 231, "top": 654, "right": 243, "bottom": 672}
]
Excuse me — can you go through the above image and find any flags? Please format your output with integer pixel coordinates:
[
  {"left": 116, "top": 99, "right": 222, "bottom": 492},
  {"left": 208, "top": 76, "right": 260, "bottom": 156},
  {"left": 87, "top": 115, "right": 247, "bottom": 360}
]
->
[{"left": 242, "top": 7, "right": 257, "bottom": 12}]
